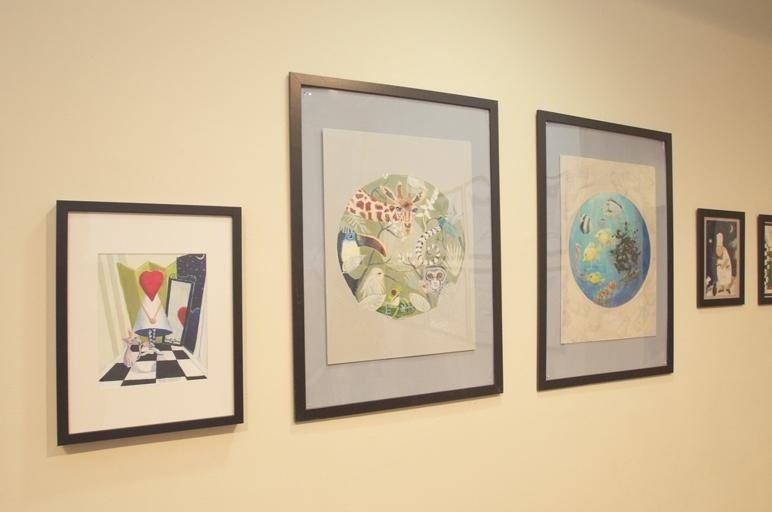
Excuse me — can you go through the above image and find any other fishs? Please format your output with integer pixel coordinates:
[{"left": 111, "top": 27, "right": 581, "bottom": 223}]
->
[{"left": 573, "top": 198, "right": 628, "bottom": 304}]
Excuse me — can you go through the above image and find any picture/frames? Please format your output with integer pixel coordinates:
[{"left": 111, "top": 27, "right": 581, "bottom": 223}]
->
[
  {"left": 537, "top": 109, "right": 673, "bottom": 392},
  {"left": 697, "top": 209, "right": 745, "bottom": 307},
  {"left": 758, "top": 215, "right": 772, "bottom": 304},
  {"left": 56, "top": 200, "right": 243, "bottom": 446},
  {"left": 289, "top": 71, "right": 503, "bottom": 422}
]
